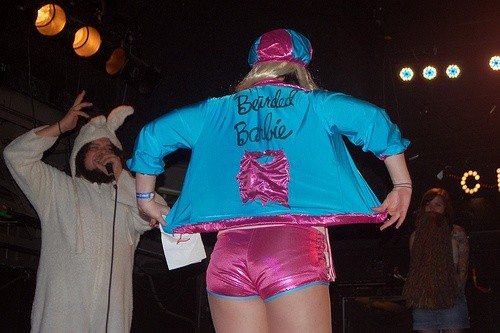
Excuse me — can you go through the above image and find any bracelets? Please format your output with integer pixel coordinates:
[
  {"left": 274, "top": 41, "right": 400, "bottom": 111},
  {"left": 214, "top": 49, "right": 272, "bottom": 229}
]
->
[
  {"left": 57, "top": 119, "right": 64, "bottom": 135},
  {"left": 392, "top": 183, "right": 412, "bottom": 188},
  {"left": 135, "top": 192, "right": 156, "bottom": 200}
]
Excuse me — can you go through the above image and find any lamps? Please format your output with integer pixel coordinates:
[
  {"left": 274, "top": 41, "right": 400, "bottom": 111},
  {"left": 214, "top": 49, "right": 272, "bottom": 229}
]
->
[
  {"left": 122, "top": 64, "right": 162, "bottom": 97},
  {"left": 66, "top": 18, "right": 105, "bottom": 60},
  {"left": 92, "top": 39, "right": 127, "bottom": 78},
  {"left": 19, "top": 0, "right": 71, "bottom": 42}
]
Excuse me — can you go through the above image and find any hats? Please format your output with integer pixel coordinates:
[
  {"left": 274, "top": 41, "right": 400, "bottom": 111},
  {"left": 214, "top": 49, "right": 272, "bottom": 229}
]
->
[
  {"left": 247, "top": 30, "right": 313, "bottom": 68},
  {"left": 70, "top": 106, "right": 134, "bottom": 254}
]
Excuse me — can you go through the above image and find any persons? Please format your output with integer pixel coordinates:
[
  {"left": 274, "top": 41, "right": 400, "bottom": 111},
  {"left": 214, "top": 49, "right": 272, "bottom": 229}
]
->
[
  {"left": 126, "top": 28, "right": 413, "bottom": 333},
  {"left": 2, "top": 91, "right": 167, "bottom": 333},
  {"left": 406, "top": 187, "right": 471, "bottom": 333}
]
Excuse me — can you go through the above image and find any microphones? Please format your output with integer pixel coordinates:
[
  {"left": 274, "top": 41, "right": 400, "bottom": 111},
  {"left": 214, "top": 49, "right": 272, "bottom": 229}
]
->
[{"left": 105, "top": 163, "right": 117, "bottom": 189}]
